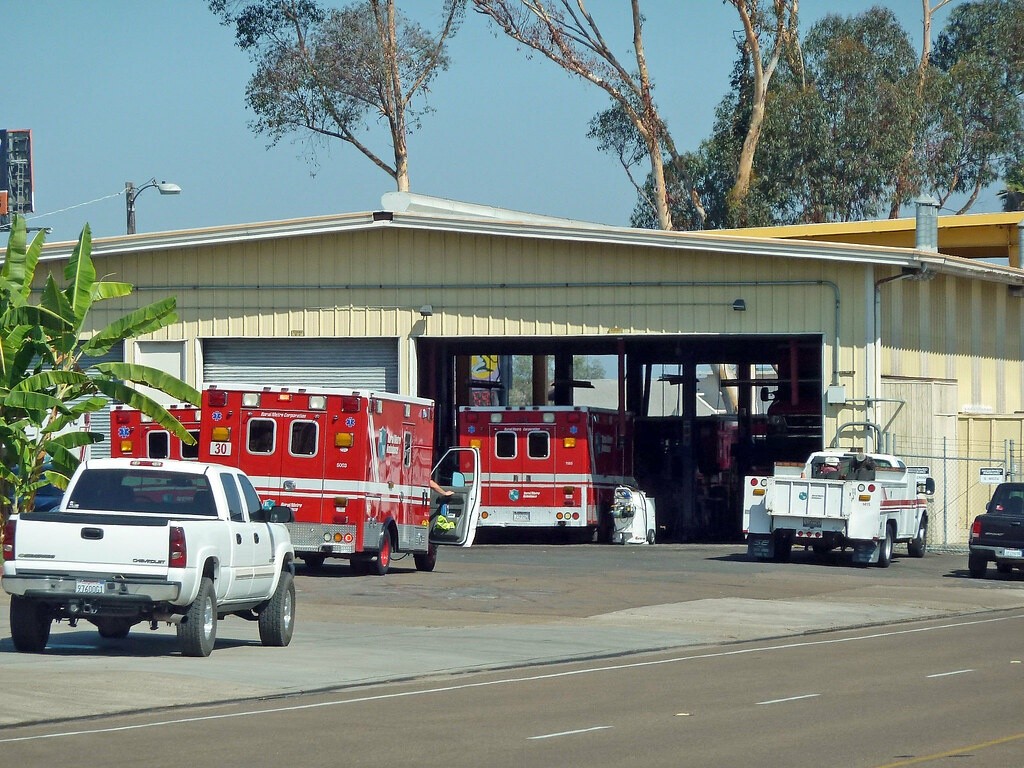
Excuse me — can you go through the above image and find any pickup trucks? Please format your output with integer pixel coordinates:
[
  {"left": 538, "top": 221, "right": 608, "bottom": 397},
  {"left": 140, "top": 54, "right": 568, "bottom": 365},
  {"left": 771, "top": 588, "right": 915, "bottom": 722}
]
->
[
  {"left": 3, "top": 457, "right": 296, "bottom": 659},
  {"left": 968, "top": 483, "right": 1024, "bottom": 577},
  {"left": 742, "top": 452, "right": 936, "bottom": 566}
]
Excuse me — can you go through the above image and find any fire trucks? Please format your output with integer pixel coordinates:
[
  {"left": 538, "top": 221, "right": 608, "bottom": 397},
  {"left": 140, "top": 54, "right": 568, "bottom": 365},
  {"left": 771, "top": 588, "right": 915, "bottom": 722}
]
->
[
  {"left": 459, "top": 405, "right": 640, "bottom": 544},
  {"left": 110, "top": 403, "right": 201, "bottom": 460},
  {"left": 199, "top": 387, "right": 481, "bottom": 575}
]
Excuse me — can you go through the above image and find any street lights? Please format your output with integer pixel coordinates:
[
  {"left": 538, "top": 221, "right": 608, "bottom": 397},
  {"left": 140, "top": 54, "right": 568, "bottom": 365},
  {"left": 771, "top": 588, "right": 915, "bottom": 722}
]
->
[{"left": 125, "top": 183, "right": 183, "bottom": 234}]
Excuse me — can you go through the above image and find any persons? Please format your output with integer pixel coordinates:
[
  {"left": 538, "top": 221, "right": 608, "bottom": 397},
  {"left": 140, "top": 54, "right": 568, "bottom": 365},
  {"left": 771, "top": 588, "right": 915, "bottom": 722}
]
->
[{"left": 429, "top": 479, "right": 455, "bottom": 496}]
